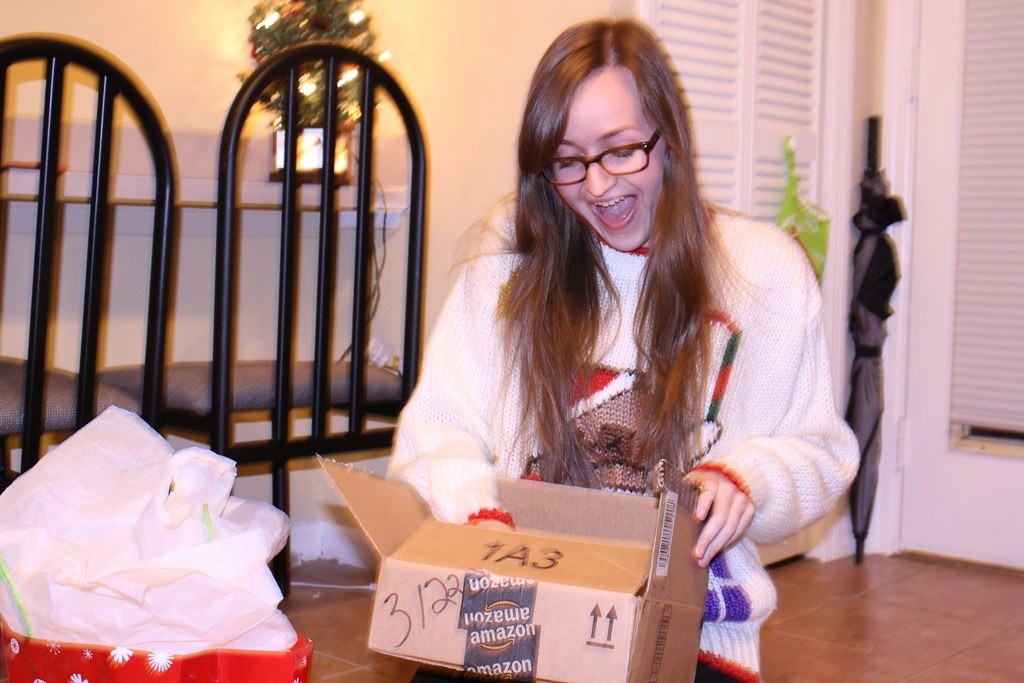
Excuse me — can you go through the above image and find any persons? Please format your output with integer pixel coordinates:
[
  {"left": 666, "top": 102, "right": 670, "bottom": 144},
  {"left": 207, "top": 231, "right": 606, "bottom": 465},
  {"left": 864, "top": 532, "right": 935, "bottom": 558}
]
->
[{"left": 386, "top": 18, "right": 862, "bottom": 572}]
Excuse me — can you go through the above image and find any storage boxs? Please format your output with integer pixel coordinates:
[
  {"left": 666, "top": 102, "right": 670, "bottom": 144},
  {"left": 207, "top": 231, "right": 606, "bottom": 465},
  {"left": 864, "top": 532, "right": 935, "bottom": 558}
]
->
[{"left": 312, "top": 453, "right": 708, "bottom": 677}]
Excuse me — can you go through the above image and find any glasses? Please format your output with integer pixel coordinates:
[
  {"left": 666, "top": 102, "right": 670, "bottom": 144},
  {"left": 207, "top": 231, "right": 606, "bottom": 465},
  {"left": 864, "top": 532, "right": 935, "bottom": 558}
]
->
[{"left": 542, "top": 127, "right": 663, "bottom": 184}]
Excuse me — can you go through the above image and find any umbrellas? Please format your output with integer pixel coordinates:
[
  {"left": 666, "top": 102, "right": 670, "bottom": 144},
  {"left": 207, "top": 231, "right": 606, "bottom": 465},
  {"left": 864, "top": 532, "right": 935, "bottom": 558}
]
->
[{"left": 844, "top": 115, "right": 906, "bottom": 566}]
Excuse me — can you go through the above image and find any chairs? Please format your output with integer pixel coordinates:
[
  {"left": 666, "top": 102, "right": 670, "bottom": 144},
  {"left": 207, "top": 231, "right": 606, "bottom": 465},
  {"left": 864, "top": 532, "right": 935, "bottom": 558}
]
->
[
  {"left": 0, "top": 33, "right": 179, "bottom": 441},
  {"left": 80, "top": 39, "right": 427, "bottom": 609}
]
